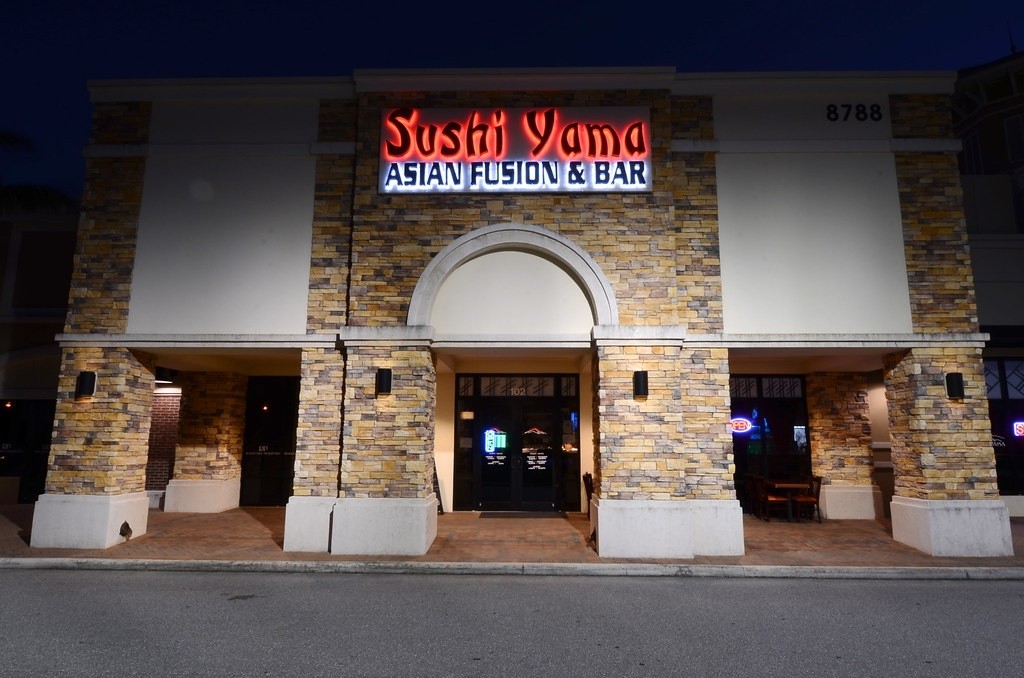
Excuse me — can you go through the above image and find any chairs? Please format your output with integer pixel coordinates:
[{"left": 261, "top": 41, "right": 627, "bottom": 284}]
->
[
  {"left": 744, "top": 471, "right": 791, "bottom": 522},
  {"left": 793, "top": 475, "right": 823, "bottom": 524}
]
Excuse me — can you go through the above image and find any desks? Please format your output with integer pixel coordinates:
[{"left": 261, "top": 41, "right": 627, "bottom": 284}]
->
[{"left": 755, "top": 477, "right": 812, "bottom": 524}]
